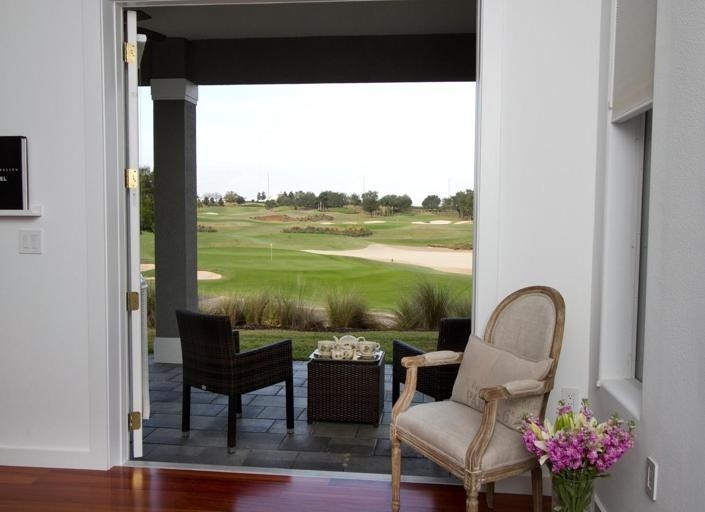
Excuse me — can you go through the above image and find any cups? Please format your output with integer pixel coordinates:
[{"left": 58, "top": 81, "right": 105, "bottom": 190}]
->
[
  {"left": 359, "top": 341, "right": 381, "bottom": 355},
  {"left": 317, "top": 340, "right": 336, "bottom": 354},
  {"left": 344, "top": 350, "right": 354, "bottom": 360},
  {"left": 330, "top": 349, "right": 347, "bottom": 360}
]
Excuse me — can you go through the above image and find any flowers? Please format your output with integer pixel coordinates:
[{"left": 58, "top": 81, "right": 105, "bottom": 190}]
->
[{"left": 516, "top": 394, "right": 638, "bottom": 512}]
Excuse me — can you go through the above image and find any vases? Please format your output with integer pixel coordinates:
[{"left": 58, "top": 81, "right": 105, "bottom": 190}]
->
[{"left": 551, "top": 472, "right": 597, "bottom": 511}]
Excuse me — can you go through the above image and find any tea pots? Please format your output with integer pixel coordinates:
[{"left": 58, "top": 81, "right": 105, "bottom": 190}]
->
[{"left": 332, "top": 335, "right": 366, "bottom": 349}]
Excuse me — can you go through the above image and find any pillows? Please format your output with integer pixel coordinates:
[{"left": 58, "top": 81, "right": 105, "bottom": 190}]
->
[{"left": 452, "top": 335, "right": 553, "bottom": 430}]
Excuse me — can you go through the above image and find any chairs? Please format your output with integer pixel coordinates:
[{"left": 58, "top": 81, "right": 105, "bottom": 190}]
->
[
  {"left": 389, "top": 287, "right": 567, "bottom": 512},
  {"left": 175, "top": 310, "right": 295, "bottom": 454},
  {"left": 392, "top": 318, "right": 470, "bottom": 413}
]
